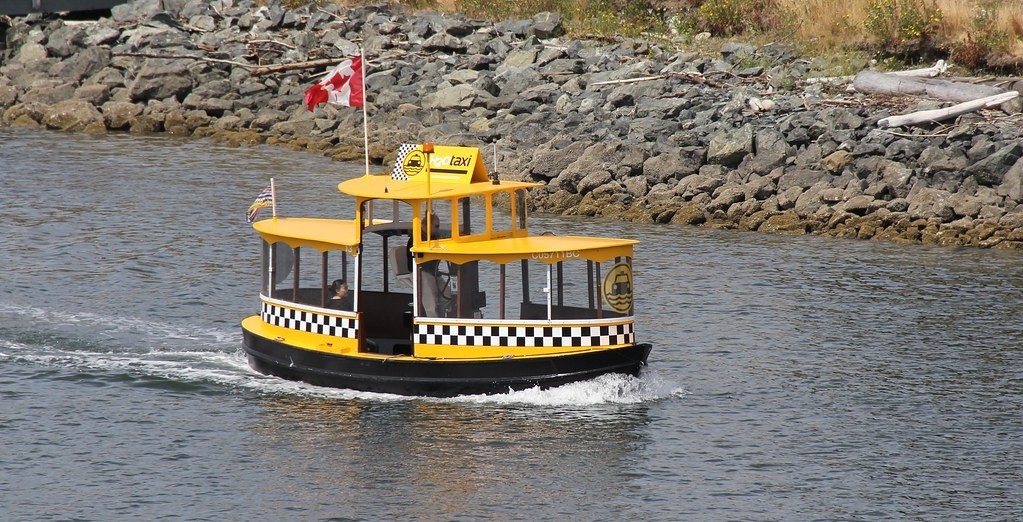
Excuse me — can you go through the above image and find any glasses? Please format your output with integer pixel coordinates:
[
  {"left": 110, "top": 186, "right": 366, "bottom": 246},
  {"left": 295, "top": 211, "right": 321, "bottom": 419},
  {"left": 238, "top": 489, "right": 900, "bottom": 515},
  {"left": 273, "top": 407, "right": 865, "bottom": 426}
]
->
[{"left": 432, "top": 223, "right": 440, "bottom": 229}]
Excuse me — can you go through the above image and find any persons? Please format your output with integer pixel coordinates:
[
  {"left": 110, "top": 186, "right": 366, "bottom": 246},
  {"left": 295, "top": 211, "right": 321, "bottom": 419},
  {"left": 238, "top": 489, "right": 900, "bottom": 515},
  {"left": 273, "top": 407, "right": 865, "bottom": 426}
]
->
[
  {"left": 328, "top": 279, "right": 352, "bottom": 311},
  {"left": 406, "top": 216, "right": 451, "bottom": 318}
]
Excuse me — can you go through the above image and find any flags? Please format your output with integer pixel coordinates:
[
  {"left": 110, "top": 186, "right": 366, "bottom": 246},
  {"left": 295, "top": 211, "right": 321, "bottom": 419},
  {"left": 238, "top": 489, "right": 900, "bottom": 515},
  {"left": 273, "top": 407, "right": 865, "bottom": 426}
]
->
[
  {"left": 304, "top": 55, "right": 364, "bottom": 112},
  {"left": 246, "top": 185, "right": 273, "bottom": 223}
]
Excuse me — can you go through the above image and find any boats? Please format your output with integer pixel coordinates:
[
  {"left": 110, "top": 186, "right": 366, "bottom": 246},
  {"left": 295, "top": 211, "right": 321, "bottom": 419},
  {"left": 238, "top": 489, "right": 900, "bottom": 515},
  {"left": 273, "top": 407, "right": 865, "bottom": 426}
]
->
[{"left": 241, "top": 143, "right": 652, "bottom": 400}]
